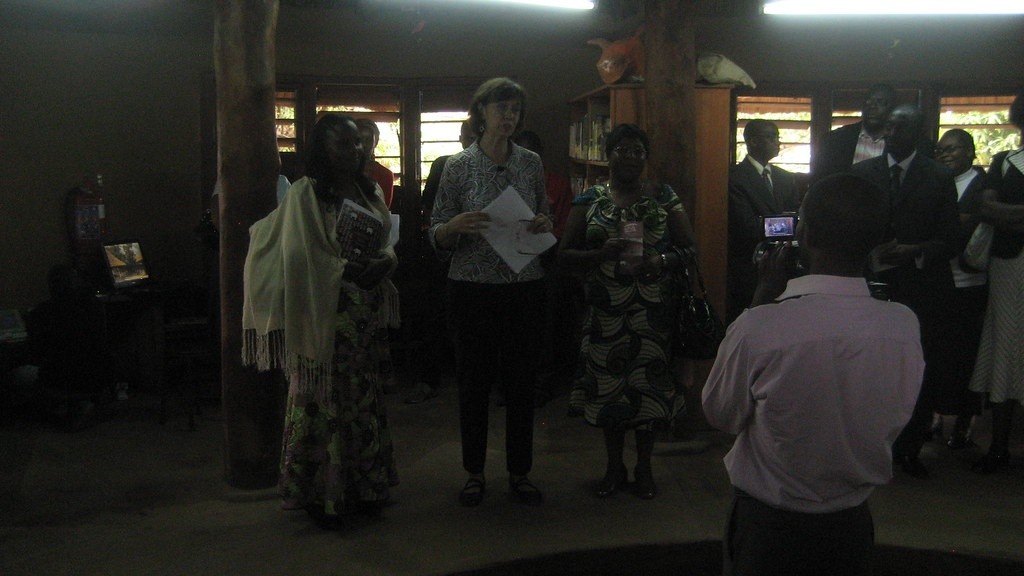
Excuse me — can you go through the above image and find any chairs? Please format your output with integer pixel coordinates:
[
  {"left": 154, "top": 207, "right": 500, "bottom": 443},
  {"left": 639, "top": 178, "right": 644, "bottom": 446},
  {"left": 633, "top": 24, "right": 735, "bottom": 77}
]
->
[{"left": 159, "top": 210, "right": 218, "bottom": 430}]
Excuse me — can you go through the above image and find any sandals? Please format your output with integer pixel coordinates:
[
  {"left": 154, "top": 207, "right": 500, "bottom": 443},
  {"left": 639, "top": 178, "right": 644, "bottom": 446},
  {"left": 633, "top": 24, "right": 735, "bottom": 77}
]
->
[
  {"left": 460, "top": 475, "right": 484, "bottom": 506},
  {"left": 509, "top": 476, "right": 541, "bottom": 506}
]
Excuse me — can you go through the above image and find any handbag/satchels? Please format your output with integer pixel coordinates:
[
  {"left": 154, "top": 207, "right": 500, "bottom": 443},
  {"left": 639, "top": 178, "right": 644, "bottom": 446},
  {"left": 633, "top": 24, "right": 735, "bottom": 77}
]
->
[
  {"left": 964, "top": 148, "right": 1017, "bottom": 271},
  {"left": 669, "top": 247, "right": 725, "bottom": 358}
]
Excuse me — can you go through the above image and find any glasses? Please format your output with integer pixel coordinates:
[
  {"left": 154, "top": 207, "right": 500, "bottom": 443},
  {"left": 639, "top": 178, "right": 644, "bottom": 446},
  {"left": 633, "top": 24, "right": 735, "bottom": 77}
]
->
[
  {"left": 865, "top": 97, "right": 891, "bottom": 107},
  {"left": 934, "top": 144, "right": 972, "bottom": 158},
  {"left": 749, "top": 134, "right": 781, "bottom": 143},
  {"left": 613, "top": 146, "right": 647, "bottom": 158}
]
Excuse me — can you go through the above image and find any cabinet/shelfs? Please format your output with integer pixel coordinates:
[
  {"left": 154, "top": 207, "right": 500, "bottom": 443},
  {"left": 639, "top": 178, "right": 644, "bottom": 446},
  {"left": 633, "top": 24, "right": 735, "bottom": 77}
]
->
[{"left": 566, "top": 83, "right": 637, "bottom": 203}]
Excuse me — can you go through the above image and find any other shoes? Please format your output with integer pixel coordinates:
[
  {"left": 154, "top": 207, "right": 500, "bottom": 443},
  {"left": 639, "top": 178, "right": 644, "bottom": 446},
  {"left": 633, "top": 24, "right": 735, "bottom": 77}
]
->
[
  {"left": 948, "top": 435, "right": 967, "bottom": 449},
  {"left": 307, "top": 502, "right": 342, "bottom": 530},
  {"left": 971, "top": 449, "right": 1010, "bottom": 476},
  {"left": 925, "top": 419, "right": 944, "bottom": 441},
  {"left": 633, "top": 463, "right": 656, "bottom": 499},
  {"left": 597, "top": 460, "right": 627, "bottom": 497},
  {"left": 901, "top": 452, "right": 923, "bottom": 477}
]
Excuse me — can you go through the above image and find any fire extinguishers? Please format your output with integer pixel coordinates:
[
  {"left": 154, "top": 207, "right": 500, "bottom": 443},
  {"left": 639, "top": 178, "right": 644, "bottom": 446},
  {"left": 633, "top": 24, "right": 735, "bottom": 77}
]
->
[{"left": 63, "top": 174, "right": 107, "bottom": 279}]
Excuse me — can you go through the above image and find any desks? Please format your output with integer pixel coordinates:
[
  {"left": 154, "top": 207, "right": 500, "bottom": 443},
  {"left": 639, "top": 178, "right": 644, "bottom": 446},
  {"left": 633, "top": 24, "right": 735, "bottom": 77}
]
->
[{"left": 45, "top": 283, "right": 171, "bottom": 434}]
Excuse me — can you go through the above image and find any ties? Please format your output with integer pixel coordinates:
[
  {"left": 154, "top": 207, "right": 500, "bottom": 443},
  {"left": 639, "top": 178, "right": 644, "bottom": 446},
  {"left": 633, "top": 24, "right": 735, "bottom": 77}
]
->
[
  {"left": 891, "top": 165, "right": 903, "bottom": 197},
  {"left": 762, "top": 169, "right": 774, "bottom": 193}
]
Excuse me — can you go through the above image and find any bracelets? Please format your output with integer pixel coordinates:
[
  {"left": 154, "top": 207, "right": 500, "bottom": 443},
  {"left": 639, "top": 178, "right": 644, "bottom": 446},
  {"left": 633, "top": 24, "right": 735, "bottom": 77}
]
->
[{"left": 661, "top": 253, "right": 667, "bottom": 272}]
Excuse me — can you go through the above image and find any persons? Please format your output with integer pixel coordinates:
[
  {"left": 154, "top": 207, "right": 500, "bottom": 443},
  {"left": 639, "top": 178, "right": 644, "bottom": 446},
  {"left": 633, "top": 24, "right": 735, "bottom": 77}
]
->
[
  {"left": 851, "top": 104, "right": 989, "bottom": 478},
  {"left": 970, "top": 90, "right": 1024, "bottom": 459},
  {"left": 700, "top": 172, "right": 927, "bottom": 576},
  {"left": 555, "top": 125, "right": 697, "bottom": 498},
  {"left": 242, "top": 117, "right": 394, "bottom": 525},
  {"left": 407, "top": 77, "right": 573, "bottom": 505},
  {"left": 729, "top": 84, "right": 894, "bottom": 305}
]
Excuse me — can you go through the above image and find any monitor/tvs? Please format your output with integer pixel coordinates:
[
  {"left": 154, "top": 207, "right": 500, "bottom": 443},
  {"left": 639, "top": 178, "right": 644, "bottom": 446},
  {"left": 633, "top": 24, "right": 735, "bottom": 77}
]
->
[{"left": 100, "top": 238, "right": 154, "bottom": 290}]
